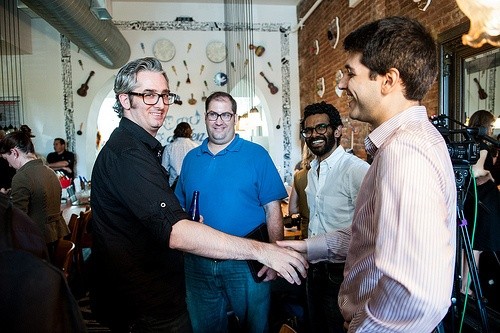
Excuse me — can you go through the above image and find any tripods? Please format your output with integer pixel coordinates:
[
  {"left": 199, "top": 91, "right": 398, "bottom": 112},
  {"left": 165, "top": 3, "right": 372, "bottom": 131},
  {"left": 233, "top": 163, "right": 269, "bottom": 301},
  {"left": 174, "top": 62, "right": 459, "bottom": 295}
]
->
[{"left": 443, "top": 180, "right": 492, "bottom": 333}]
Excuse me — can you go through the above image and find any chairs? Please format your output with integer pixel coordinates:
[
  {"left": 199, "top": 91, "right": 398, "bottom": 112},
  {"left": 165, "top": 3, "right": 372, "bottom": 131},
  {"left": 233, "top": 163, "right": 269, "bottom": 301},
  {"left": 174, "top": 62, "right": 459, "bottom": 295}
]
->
[{"left": 64, "top": 206, "right": 91, "bottom": 289}]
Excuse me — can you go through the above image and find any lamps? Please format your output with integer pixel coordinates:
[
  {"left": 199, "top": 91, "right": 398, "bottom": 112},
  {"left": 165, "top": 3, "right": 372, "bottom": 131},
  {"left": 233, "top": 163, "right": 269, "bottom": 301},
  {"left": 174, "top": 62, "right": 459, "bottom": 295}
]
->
[{"left": 90, "top": 0, "right": 114, "bottom": 20}]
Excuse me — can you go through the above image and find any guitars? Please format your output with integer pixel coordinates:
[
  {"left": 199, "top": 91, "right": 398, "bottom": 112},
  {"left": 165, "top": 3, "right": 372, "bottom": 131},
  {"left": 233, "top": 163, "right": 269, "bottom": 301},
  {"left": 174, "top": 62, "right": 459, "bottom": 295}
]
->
[
  {"left": 260, "top": 72, "right": 279, "bottom": 95},
  {"left": 77, "top": 71, "right": 95, "bottom": 97}
]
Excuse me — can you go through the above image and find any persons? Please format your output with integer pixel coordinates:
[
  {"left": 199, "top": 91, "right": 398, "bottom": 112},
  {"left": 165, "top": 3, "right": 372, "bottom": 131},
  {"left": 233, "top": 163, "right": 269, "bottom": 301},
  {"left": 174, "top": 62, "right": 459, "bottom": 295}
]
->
[
  {"left": 46, "top": 138, "right": 76, "bottom": 188},
  {"left": 89, "top": 58, "right": 309, "bottom": 333},
  {"left": 257, "top": 17, "right": 458, "bottom": 333},
  {"left": 174, "top": 92, "right": 288, "bottom": 333},
  {"left": 0, "top": 129, "right": 16, "bottom": 193},
  {"left": 0, "top": 125, "right": 72, "bottom": 279},
  {"left": 301, "top": 103, "right": 372, "bottom": 333},
  {"left": 289, "top": 143, "right": 312, "bottom": 240},
  {"left": 0, "top": 157, "right": 87, "bottom": 333},
  {"left": 459, "top": 110, "right": 499, "bottom": 304},
  {"left": 19, "top": 126, "right": 35, "bottom": 140},
  {"left": 162, "top": 121, "right": 203, "bottom": 187}
]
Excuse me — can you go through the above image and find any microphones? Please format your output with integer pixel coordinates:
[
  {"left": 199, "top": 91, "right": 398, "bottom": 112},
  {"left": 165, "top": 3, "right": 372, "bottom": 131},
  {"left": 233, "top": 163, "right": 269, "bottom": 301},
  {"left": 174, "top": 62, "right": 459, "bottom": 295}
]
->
[{"left": 463, "top": 127, "right": 487, "bottom": 136}]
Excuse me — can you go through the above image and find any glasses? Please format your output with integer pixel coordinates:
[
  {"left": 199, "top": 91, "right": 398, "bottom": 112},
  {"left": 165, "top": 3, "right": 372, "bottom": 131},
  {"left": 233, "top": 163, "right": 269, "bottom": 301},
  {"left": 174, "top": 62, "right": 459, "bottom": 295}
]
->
[
  {"left": 300, "top": 124, "right": 335, "bottom": 138},
  {"left": 206, "top": 111, "right": 235, "bottom": 122},
  {"left": 124, "top": 92, "right": 177, "bottom": 105}
]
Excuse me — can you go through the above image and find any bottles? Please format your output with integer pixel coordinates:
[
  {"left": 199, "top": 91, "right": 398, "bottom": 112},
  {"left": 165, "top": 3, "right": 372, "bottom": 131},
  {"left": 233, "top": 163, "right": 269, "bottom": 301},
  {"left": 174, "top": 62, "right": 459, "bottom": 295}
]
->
[{"left": 187, "top": 191, "right": 200, "bottom": 223}]
